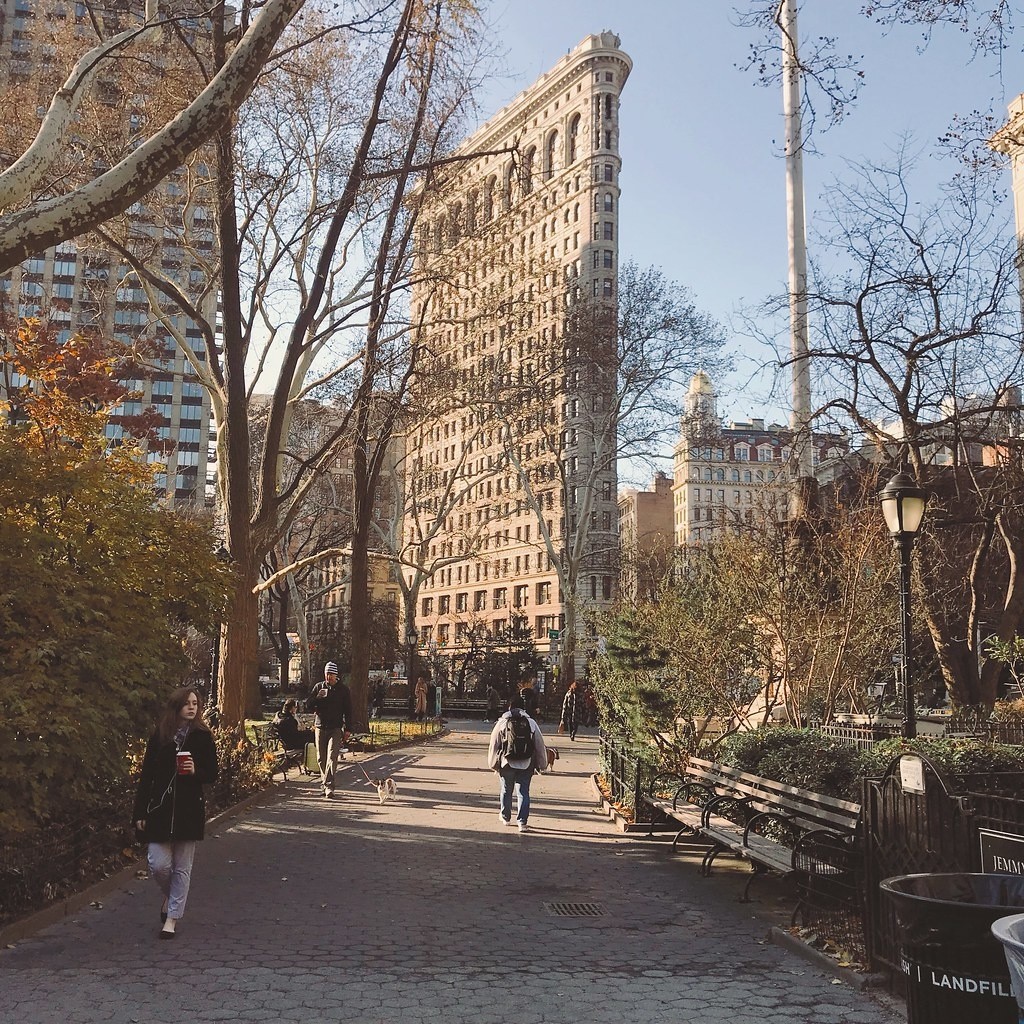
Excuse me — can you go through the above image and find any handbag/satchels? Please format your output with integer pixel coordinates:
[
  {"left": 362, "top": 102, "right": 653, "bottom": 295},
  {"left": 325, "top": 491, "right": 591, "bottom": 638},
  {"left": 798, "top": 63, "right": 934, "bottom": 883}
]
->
[{"left": 558, "top": 718, "right": 564, "bottom": 734}]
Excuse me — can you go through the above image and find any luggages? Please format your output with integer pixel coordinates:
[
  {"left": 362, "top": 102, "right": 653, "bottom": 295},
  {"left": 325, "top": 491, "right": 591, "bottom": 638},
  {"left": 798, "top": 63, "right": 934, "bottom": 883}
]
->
[{"left": 304, "top": 742, "right": 321, "bottom": 776}]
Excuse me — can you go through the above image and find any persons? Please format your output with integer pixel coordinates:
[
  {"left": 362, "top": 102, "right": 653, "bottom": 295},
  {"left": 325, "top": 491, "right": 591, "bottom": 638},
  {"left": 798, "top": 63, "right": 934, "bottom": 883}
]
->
[
  {"left": 304, "top": 661, "right": 352, "bottom": 798},
  {"left": 520, "top": 676, "right": 544, "bottom": 776},
  {"left": 560, "top": 678, "right": 596, "bottom": 740},
  {"left": 488, "top": 695, "right": 548, "bottom": 833},
  {"left": 273, "top": 698, "right": 315, "bottom": 751},
  {"left": 482, "top": 682, "right": 499, "bottom": 723},
  {"left": 132, "top": 687, "right": 217, "bottom": 941},
  {"left": 367, "top": 674, "right": 388, "bottom": 720},
  {"left": 415, "top": 677, "right": 436, "bottom": 722}
]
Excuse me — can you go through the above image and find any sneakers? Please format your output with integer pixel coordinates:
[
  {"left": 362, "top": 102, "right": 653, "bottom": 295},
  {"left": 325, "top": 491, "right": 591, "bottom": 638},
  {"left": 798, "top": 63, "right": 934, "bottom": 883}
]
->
[
  {"left": 499, "top": 812, "right": 511, "bottom": 825},
  {"left": 325, "top": 789, "right": 335, "bottom": 798},
  {"left": 518, "top": 823, "right": 528, "bottom": 832}
]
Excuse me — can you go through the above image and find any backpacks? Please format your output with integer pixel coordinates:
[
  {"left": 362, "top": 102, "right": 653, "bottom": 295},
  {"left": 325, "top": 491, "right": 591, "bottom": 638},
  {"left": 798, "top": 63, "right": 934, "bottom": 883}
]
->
[{"left": 499, "top": 715, "right": 535, "bottom": 760}]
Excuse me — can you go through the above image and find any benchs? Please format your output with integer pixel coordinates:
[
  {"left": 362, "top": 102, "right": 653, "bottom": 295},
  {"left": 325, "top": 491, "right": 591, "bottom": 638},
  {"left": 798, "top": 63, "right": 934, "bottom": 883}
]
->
[
  {"left": 641, "top": 757, "right": 863, "bottom": 931},
  {"left": 383, "top": 698, "right": 508, "bottom": 720},
  {"left": 339, "top": 716, "right": 370, "bottom": 760},
  {"left": 252, "top": 716, "right": 315, "bottom": 782}
]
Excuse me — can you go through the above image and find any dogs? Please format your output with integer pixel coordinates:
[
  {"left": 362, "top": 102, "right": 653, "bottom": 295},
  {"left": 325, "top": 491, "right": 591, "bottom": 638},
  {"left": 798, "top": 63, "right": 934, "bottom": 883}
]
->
[
  {"left": 534, "top": 748, "right": 559, "bottom": 775},
  {"left": 373, "top": 778, "right": 397, "bottom": 805}
]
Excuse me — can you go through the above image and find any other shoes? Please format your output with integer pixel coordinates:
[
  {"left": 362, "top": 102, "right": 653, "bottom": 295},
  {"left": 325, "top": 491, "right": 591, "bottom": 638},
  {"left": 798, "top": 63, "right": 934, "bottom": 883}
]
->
[
  {"left": 160, "top": 899, "right": 167, "bottom": 923},
  {"left": 160, "top": 926, "right": 176, "bottom": 938},
  {"left": 571, "top": 732, "right": 574, "bottom": 741}
]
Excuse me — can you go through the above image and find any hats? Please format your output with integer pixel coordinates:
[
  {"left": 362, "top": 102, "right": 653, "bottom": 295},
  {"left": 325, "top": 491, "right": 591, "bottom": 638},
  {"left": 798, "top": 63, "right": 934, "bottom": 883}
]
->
[{"left": 325, "top": 662, "right": 338, "bottom": 674}]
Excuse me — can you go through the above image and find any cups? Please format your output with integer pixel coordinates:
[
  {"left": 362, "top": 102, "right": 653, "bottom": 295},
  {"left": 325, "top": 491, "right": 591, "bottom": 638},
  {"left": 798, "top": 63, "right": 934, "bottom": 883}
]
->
[
  {"left": 321, "top": 689, "right": 328, "bottom": 697},
  {"left": 176, "top": 752, "right": 191, "bottom": 775}
]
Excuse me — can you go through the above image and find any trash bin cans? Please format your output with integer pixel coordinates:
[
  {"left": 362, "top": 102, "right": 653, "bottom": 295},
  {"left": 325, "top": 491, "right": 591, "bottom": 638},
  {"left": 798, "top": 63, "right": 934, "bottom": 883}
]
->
[
  {"left": 989, "top": 912, "right": 1023, "bottom": 1023},
  {"left": 876, "top": 870, "right": 1024, "bottom": 1023}
]
